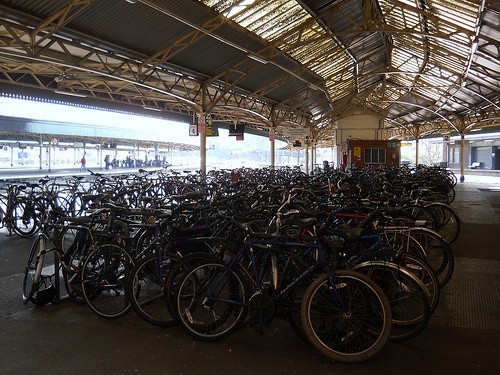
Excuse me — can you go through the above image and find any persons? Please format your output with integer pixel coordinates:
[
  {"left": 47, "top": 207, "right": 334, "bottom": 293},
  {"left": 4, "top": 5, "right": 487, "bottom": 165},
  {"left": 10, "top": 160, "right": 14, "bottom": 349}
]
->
[
  {"left": 104, "top": 154, "right": 169, "bottom": 169},
  {"left": 81, "top": 156, "right": 87, "bottom": 171}
]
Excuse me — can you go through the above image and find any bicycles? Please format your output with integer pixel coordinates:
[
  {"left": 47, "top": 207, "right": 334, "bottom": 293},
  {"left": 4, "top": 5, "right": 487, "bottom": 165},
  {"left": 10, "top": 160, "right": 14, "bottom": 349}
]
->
[{"left": 0, "top": 162, "right": 463, "bottom": 362}]
